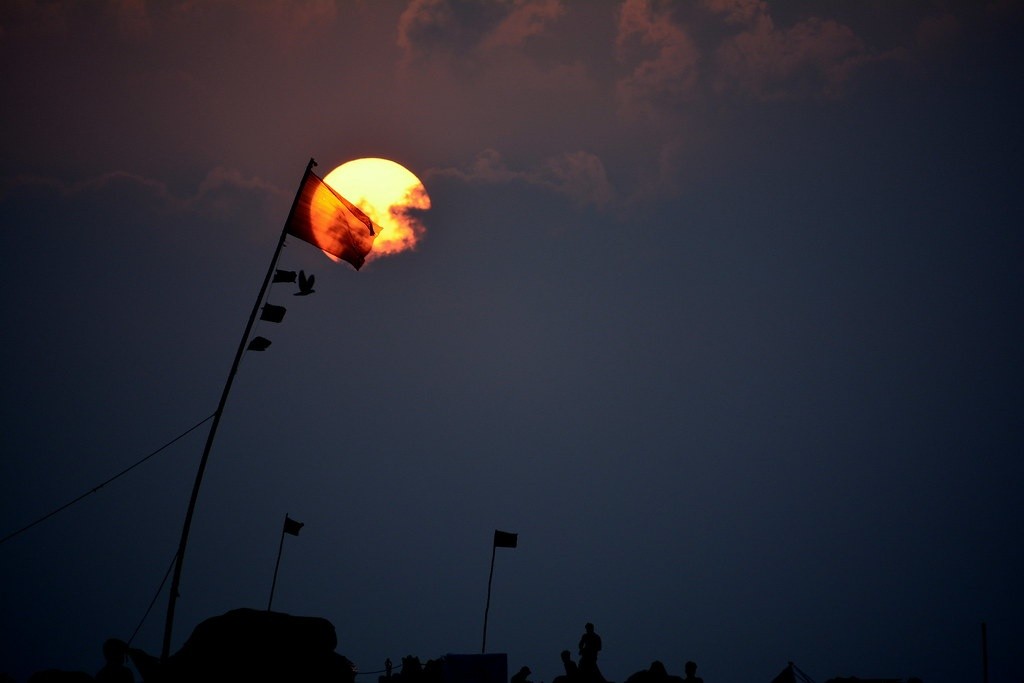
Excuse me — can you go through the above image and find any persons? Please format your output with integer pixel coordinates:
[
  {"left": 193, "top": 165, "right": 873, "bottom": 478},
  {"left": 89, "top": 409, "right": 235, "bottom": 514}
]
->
[
  {"left": 650, "top": 660, "right": 707, "bottom": 683},
  {"left": 96, "top": 638, "right": 138, "bottom": 683},
  {"left": 560, "top": 624, "right": 608, "bottom": 683},
  {"left": 511, "top": 667, "right": 530, "bottom": 683},
  {"left": 384, "top": 658, "right": 393, "bottom": 678},
  {"left": 397, "top": 655, "right": 423, "bottom": 677}
]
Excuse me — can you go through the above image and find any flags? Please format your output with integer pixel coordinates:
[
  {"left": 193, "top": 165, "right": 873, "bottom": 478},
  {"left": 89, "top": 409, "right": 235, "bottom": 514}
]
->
[
  {"left": 272, "top": 270, "right": 298, "bottom": 283},
  {"left": 248, "top": 336, "right": 273, "bottom": 353},
  {"left": 495, "top": 530, "right": 518, "bottom": 548},
  {"left": 260, "top": 302, "right": 287, "bottom": 323},
  {"left": 284, "top": 516, "right": 304, "bottom": 536},
  {"left": 284, "top": 171, "right": 384, "bottom": 271}
]
